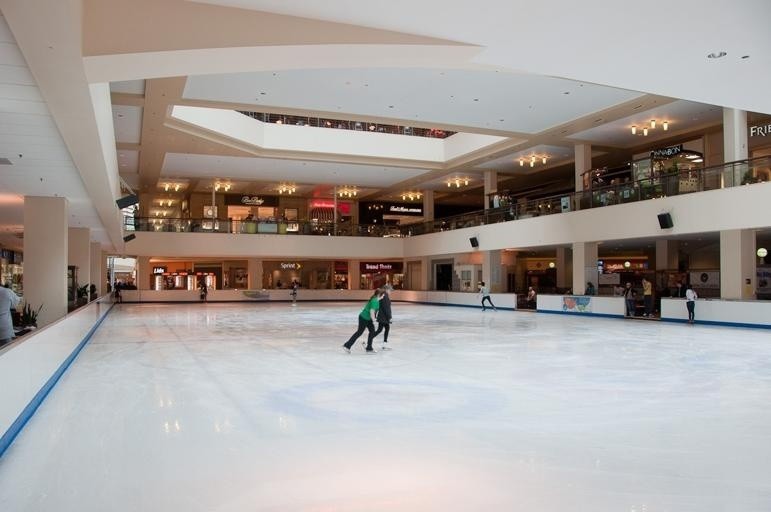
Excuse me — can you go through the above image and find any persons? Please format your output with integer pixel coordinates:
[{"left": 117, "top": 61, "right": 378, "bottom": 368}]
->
[
  {"left": 114, "top": 282, "right": 122, "bottom": 303},
  {"left": 479, "top": 282, "right": 497, "bottom": 312},
  {"left": 685, "top": 285, "right": 698, "bottom": 322},
  {"left": 614, "top": 277, "right": 653, "bottom": 316},
  {"left": 133, "top": 206, "right": 140, "bottom": 231},
  {"left": 246, "top": 214, "right": 258, "bottom": 222},
  {"left": 276, "top": 280, "right": 301, "bottom": 306},
  {"left": 585, "top": 282, "right": 594, "bottom": 295},
  {"left": 527, "top": 286, "right": 535, "bottom": 302},
  {"left": 363, "top": 285, "right": 394, "bottom": 349},
  {"left": 343, "top": 288, "right": 386, "bottom": 354},
  {"left": 670, "top": 280, "right": 685, "bottom": 298},
  {"left": 199, "top": 278, "right": 207, "bottom": 303},
  {"left": 500, "top": 195, "right": 517, "bottom": 221},
  {"left": 745, "top": 279, "right": 753, "bottom": 299},
  {"left": 0, "top": 285, "right": 24, "bottom": 346}
]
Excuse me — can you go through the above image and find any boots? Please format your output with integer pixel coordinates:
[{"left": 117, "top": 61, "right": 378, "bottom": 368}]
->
[{"left": 342, "top": 341, "right": 392, "bottom": 353}]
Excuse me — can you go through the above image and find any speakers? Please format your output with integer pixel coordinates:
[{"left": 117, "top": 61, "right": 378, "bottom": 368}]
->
[
  {"left": 658, "top": 214, "right": 673, "bottom": 228},
  {"left": 470, "top": 237, "right": 478, "bottom": 247},
  {"left": 116, "top": 194, "right": 139, "bottom": 209},
  {"left": 123, "top": 234, "right": 136, "bottom": 242}
]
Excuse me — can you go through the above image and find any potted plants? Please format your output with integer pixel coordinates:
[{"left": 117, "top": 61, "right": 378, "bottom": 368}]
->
[{"left": 68, "top": 284, "right": 91, "bottom": 305}]
